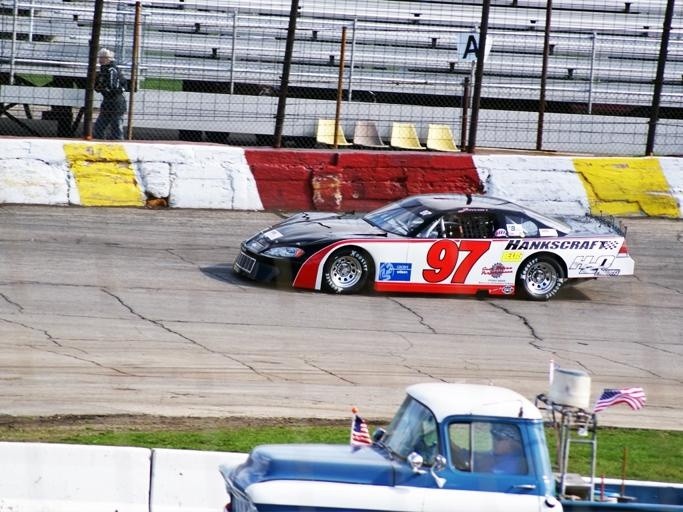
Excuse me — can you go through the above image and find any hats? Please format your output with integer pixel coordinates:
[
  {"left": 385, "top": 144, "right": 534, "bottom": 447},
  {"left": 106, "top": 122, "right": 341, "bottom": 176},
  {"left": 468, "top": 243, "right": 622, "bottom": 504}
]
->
[
  {"left": 98, "top": 49, "right": 117, "bottom": 62},
  {"left": 490, "top": 423, "right": 521, "bottom": 442}
]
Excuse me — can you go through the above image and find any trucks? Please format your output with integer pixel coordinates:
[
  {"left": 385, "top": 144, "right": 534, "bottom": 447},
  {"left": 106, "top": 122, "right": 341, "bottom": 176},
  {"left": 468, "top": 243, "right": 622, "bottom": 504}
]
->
[{"left": 219, "top": 382, "right": 683, "bottom": 512}]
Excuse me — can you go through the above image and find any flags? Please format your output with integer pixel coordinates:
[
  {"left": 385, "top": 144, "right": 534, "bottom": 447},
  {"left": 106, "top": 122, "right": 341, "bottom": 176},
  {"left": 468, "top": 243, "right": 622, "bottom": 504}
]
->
[
  {"left": 594, "top": 386, "right": 648, "bottom": 411},
  {"left": 350, "top": 414, "right": 371, "bottom": 445}
]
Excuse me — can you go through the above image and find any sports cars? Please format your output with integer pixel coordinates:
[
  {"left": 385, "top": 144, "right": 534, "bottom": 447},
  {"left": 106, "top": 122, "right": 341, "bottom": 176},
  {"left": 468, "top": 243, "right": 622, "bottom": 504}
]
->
[{"left": 232, "top": 193, "right": 635, "bottom": 300}]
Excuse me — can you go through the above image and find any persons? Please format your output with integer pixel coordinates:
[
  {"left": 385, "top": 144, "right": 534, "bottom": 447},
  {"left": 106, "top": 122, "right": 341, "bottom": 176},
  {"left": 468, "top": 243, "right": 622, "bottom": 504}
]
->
[
  {"left": 94, "top": 49, "right": 128, "bottom": 141},
  {"left": 449, "top": 423, "right": 526, "bottom": 475}
]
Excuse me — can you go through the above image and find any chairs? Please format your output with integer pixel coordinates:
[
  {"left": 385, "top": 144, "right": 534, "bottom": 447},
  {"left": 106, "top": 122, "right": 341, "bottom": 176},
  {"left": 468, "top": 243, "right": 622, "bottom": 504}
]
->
[{"left": 317, "top": 118, "right": 462, "bottom": 153}]
